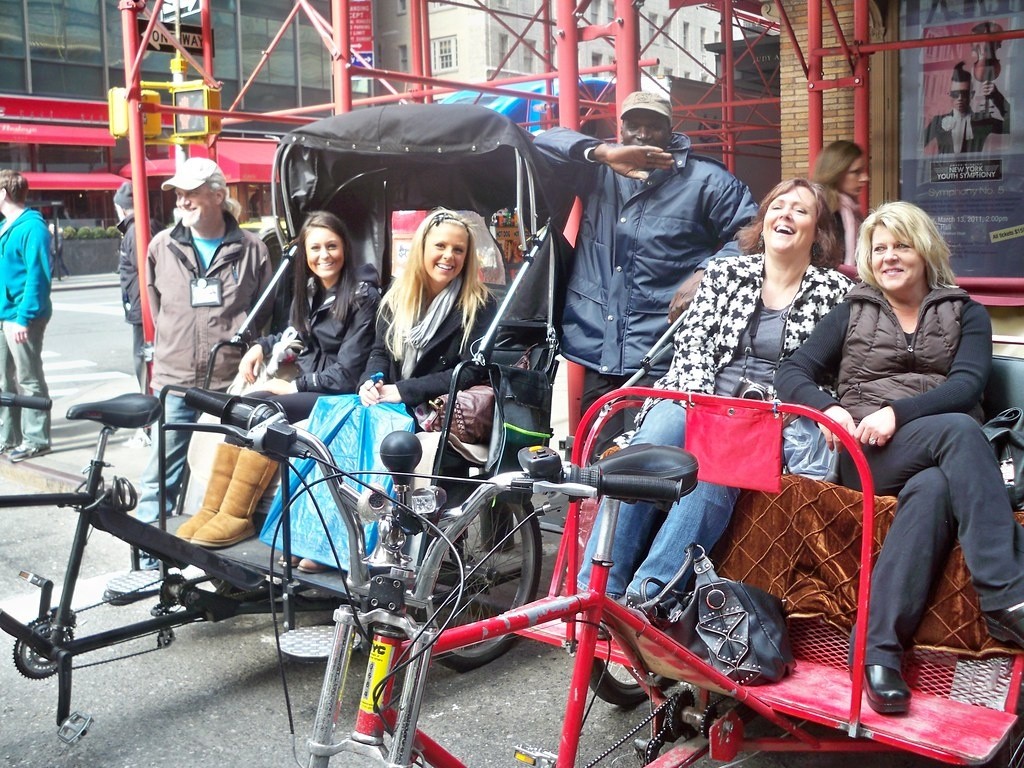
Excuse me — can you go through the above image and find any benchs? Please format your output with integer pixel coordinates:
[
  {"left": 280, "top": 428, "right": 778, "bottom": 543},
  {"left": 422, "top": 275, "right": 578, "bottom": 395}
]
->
[
  {"left": 601, "top": 353, "right": 1024, "bottom": 662},
  {"left": 202, "top": 282, "right": 542, "bottom": 470}
]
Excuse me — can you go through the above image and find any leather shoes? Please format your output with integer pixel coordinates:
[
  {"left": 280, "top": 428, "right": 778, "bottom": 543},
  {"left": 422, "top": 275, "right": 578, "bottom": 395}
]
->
[
  {"left": 983, "top": 605, "right": 1024, "bottom": 648},
  {"left": 849, "top": 663, "right": 911, "bottom": 713}
]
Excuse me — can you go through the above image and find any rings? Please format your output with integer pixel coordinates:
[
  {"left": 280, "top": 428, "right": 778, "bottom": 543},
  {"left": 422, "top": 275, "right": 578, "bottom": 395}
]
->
[
  {"left": 379, "top": 394, "right": 384, "bottom": 399},
  {"left": 368, "top": 385, "right": 373, "bottom": 391},
  {"left": 869, "top": 438, "right": 876, "bottom": 444}
]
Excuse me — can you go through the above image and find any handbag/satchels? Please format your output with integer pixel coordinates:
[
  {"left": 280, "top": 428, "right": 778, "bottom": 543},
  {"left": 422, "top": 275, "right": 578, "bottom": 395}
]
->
[
  {"left": 626, "top": 538, "right": 798, "bottom": 686},
  {"left": 982, "top": 407, "right": 1024, "bottom": 512},
  {"left": 426, "top": 343, "right": 539, "bottom": 445},
  {"left": 782, "top": 414, "right": 840, "bottom": 486},
  {"left": 227, "top": 326, "right": 305, "bottom": 396}
]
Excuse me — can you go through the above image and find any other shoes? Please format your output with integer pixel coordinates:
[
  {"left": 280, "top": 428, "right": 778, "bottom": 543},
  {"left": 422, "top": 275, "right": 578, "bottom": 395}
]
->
[
  {"left": 279, "top": 552, "right": 301, "bottom": 566},
  {"left": 298, "top": 557, "right": 327, "bottom": 573},
  {"left": 596, "top": 594, "right": 627, "bottom": 639}
]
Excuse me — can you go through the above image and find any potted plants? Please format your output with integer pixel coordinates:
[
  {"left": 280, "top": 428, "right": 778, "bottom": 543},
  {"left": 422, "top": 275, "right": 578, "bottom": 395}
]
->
[{"left": 52, "top": 226, "right": 123, "bottom": 276}]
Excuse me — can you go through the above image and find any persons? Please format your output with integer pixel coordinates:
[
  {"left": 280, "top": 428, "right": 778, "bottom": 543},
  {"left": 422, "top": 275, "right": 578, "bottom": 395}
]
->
[
  {"left": 113, "top": 182, "right": 165, "bottom": 449},
  {"left": 277, "top": 209, "right": 497, "bottom": 574},
  {"left": 49, "top": 224, "right": 70, "bottom": 279},
  {"left": 0, "top": 168, "right": 54, "bottom": 462},
  {"left": 774, "top": 202, "right": 1023, "bottom": 713},
  {"left": 137, "top": 157, "right": 272, "bottom": 572},
  {"left": 532, "top": 91, "right": 760, "bottom": 465},
  {"left": 174, "top": 211, "right": 382, "bottom": 547},
  {"left": 923, "top": 61, "right": 1010, "bottom": 154},
  {"left": 812, "top": 140, "right": 869, "bottom": 266},
  {"left": 576, "top": 176, "right": 856, "bottom": 639}
]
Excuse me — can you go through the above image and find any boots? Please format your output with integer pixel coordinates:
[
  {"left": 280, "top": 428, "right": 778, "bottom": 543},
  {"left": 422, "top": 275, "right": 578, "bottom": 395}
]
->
[
  {"left": 191, "top": 447, "right": 280, "bottom": 547},
  {"left": 174, "top": 441, "right": 244, "bottom": 542}
]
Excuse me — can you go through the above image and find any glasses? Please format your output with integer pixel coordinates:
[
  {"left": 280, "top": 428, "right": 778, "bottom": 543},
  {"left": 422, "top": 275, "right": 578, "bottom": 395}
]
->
[
  {"left": 841, "top": 166, "right": 866, "bottom": 176},
  {"left": 949, "top": 90, "right": 971, "bottom": 101}
]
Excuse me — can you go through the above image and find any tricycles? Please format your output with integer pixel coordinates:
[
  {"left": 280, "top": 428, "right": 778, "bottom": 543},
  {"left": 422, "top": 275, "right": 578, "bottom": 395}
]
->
[
  {"left": 2, "top": 108, "right": 576, "bottom": 742},
  {"left": 163, "top": 271, "right": 1024, "bottom": 768}
]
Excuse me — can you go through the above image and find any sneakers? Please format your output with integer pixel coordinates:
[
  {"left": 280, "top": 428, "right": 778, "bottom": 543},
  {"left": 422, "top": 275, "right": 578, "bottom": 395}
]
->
[
  {"left": 0, "top": 443, "right": 6, "bottom": 455},
  {"left": 10, "top": 444, "right": 51, "bottom": 461}
]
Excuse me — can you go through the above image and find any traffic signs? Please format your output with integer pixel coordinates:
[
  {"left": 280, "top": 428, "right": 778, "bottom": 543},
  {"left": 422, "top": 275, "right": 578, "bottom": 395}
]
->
[{"left": 136, "top": 16, "right": 217, "bottom": 59}]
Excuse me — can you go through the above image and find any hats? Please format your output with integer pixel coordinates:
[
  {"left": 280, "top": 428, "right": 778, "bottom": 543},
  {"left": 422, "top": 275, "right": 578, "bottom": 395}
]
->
[
  {"left": 621, "top": 91, "right": 672, "bottom": 129},
  {"left": 161, "top": 157, "right": 227, "bottom": 191},
  {"left": 113, "top": 182, "right": 134, "bottom": 209}
]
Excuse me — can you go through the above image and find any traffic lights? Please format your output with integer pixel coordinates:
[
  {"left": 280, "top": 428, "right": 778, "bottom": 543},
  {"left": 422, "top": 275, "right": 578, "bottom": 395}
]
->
[{"left": 167, "top": 81, "right": 222, "bottom": 143}]
